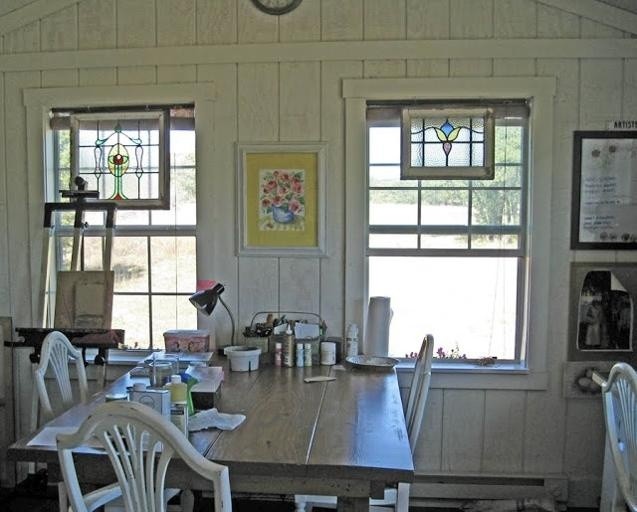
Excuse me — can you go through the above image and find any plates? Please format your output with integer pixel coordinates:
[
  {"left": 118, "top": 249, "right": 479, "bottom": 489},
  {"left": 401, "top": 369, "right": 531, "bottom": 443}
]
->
[{"left": 344, "top": 354, "right": 399, "bottom": 370}]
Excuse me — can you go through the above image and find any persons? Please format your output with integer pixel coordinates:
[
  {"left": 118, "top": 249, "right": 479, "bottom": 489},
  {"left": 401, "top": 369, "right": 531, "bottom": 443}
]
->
[{"left": 581, "top": 286, "right": 632, "bottom": 350}]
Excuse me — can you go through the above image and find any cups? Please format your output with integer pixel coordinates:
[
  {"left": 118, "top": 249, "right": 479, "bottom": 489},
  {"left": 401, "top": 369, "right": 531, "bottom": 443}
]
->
[
  {"left": 148, "top": 361, "right": 172, "bottom": 385},
  {"left": 152, "top": 352, "right": 180, "bottom": 387}
]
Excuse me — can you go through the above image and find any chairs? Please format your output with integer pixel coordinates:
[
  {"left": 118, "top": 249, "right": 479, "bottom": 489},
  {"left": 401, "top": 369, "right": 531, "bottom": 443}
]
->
[
  {"left": 591, "top": 361, "right": 636, "bottom": 512},
  {"left": 33, "top": 328, "right": 89, "bottom": 510},
  {"left": 291, "top": 331, "right": 436, "bottom": 512},
  {"left": 50, "top": 398, "right": 234, "bottom": 512}
]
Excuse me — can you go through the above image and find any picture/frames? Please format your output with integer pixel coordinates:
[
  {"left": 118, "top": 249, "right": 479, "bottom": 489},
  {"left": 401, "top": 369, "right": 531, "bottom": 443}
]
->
[
  {"left": 232, "top": 141, "right": 330, "bottom": 258},
  {"left": 569, "top": 128, "right": 637, "bottom": 251}
]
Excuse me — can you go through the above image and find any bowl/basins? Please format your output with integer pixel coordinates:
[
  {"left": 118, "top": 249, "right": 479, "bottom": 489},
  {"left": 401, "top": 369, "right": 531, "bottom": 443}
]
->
[{"left": 223, "top": 345, "right": 263, "bottom": 373}]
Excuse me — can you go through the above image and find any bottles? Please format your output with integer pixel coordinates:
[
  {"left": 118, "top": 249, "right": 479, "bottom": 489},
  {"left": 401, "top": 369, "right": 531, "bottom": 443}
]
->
[
  {"left": 346, "top": 323, "right": 358, "bottom": 356},
  {"left": 163, "top": 374, "right": 189, "bottom": 442},
  {"left": 273, "top": 323, "right": 336, "bottom": 368}
]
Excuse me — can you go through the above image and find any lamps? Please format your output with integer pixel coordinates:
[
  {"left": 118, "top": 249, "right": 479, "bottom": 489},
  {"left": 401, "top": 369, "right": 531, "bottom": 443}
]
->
[{"left": 188, "top": 281, "right": 240, "bottom": 357}]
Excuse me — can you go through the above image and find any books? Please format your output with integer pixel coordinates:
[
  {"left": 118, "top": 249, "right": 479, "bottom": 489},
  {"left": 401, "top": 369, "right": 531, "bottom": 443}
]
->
[{"left": 128, "top": 348, "right": 213, "bottom": 378}]
